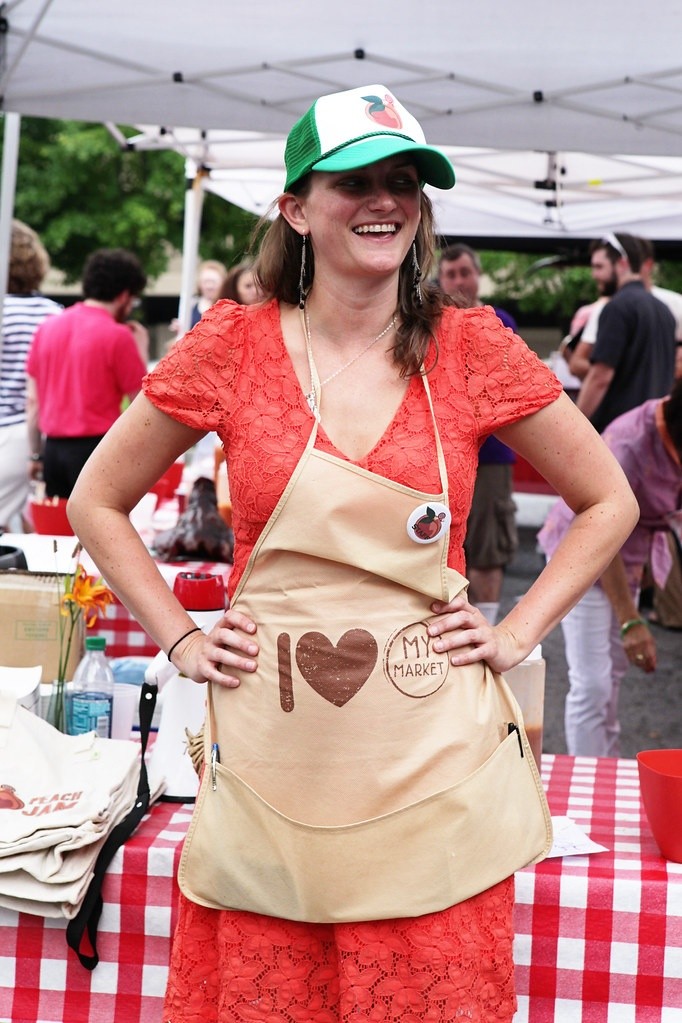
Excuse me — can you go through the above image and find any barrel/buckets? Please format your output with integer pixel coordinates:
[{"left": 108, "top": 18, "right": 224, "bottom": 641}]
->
[{"left": 147, "top": 462, "right": 184, "bottom": 510}]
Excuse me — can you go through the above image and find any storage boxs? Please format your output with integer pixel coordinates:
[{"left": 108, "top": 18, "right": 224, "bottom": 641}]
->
[{"left": 0, "top": 568, "right": 84, "bottom": 684}]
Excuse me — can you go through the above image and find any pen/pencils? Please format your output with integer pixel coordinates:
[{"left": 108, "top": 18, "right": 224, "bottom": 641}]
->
[
  {"left": 212, "top": 742, "right": 222, "bottom": 785},
  {"left": 509, "top": 722, "right": 524, "bottom": 758}
]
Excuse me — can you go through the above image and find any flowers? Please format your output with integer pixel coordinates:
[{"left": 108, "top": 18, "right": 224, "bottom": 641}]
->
[{"left": 53, "top": 540, "right": 116, "bottom": 732}]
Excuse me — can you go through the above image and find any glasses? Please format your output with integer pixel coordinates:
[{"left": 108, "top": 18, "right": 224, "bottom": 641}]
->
[{"left": 600, "top": 232, "right": 628, "bottom": 263}]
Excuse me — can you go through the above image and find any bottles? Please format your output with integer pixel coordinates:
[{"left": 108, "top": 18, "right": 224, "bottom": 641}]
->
[
  {"left": 66, "top": 636, "right": 115, "bottom": 739},
  {"left": 500, "top": 644, "right": 546, "bottom": 777}
]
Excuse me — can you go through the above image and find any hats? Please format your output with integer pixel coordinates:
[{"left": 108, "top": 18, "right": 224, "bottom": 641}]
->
[{"left": 284, "top": 84, "right": 455, "bottom": 190}]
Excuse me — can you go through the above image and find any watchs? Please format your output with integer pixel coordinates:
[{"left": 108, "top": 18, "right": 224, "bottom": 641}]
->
[{"left": 28, "top": 453, "right": 43, "bottom": 460}]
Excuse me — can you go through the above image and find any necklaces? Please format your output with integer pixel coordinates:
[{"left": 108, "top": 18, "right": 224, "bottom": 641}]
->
[{"left": 305, "top": 302, "right": 397, "bottom": 422}]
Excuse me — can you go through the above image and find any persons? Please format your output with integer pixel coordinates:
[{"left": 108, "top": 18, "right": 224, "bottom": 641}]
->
[
  {"left": 219, "top": 257, "right": 269, "bottom": 304},
  {"left": 534, "top": 376, "right": 682, "bottom": 757},
  {"left": 0, "top": 217, "right": 64, "bottom": 535},
  {"left": 439, "top": 245, "right": 517, "bottom": 624},
  {"left": 65, "top": 83, "right": 641, "bottom": 1023},
  {"left": 560, "top": 232, "right": 682, "bottom": 431},
  {"left": 29, "top": 249, "right": 146, "bottom": 502},
  {"left": 168, "top": 260, "right": 226, "bottom": 334}
]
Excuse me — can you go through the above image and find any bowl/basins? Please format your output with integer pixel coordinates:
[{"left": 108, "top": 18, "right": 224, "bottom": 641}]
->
[
  {"left": 30, "top": 498, "right": 76, "bottom": 536},
  {"left": 636, "top": 747, "right": 682, "bottom": 864},
  {"left": 172, "top": 571, "right": 226, "bottom": 611}
]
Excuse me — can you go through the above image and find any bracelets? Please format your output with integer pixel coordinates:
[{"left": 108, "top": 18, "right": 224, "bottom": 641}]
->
[
  {"left": 619, "top": 618, "right": 647, "bottom": 640},
  {"left": 168, "top": 628, "right": 201, "bottom": 662}
]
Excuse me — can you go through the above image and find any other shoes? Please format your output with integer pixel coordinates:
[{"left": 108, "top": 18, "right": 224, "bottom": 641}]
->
[{"left": 646, "top": 612, "right": 681, "bottom": 633}]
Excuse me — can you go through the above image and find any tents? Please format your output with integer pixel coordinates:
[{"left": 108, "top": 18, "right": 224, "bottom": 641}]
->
[{"left": 2, "top": 1, "right": 682, "bottom": 333}]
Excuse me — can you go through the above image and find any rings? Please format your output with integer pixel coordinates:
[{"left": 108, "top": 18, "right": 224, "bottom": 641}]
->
[{"left": 636, "top": 655, "right": 643, "bottom": 660}]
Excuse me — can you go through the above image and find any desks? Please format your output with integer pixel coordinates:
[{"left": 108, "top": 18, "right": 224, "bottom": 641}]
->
[
  {"left": 514, "top": 358, "right": 581, "bottom": 483},
  {"left": 1, "top": 731, "right": 682, "bottom": 1023},
  {"left": 86, "top": 559, "right": 233, "bottom": 658}
]
